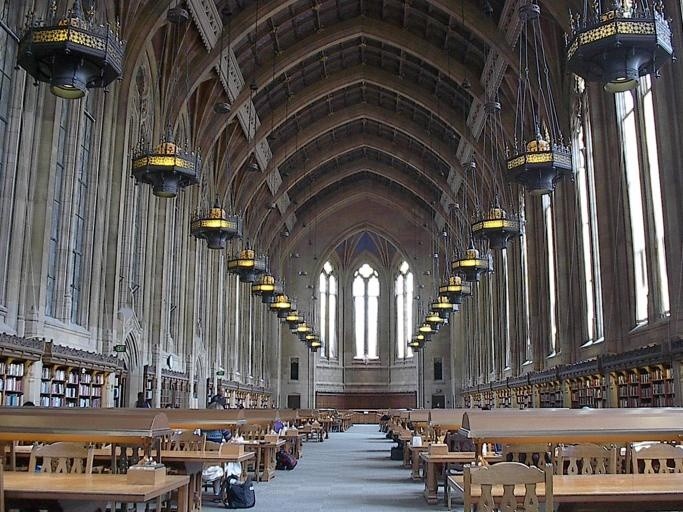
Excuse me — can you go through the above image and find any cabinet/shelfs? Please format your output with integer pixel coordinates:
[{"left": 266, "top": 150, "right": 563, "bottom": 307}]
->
[
  {"left": 0, "top": 344, "right": 274, "bottom": 409},
  {"left": 464, "top": 361, "right": 675, "bottom": 410}
]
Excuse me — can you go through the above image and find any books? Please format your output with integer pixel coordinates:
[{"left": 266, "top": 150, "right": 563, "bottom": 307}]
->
[
  {"left": 225, "top": 389, "right": 268, "bottom": 409},
  {"left": 207, "top": 388, "right": 211, "bottom": 403},
  {"left": 464, "top": 365, "right": 676, "bottom": 408},
  {"left": 0, "top": 358, "right": 124, "bottom": 409},
  {"left": 147, "top": 374, "right": 189, "bottom": 408}
]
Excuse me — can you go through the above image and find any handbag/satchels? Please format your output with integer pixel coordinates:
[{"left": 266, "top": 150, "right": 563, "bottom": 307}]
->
[
  {"left": 220, "top": 475, "right": 255, "bottom": 508},
  {"left": 276, "top": 450, "right": 297, "bottom": 470}
]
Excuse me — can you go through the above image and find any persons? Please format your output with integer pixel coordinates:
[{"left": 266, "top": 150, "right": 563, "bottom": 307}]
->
[
  {"left": 273, "top": 417, "right": 283, "bottom": 433},
  {"left": 133, "top": 392, "right": 151, "bottom": 408},
  {"left": 211, "top": 386, "right": 226, "bottom": 408},
  {"left": 198, "top": 402, "right": 232, "bottom": 495}
]
